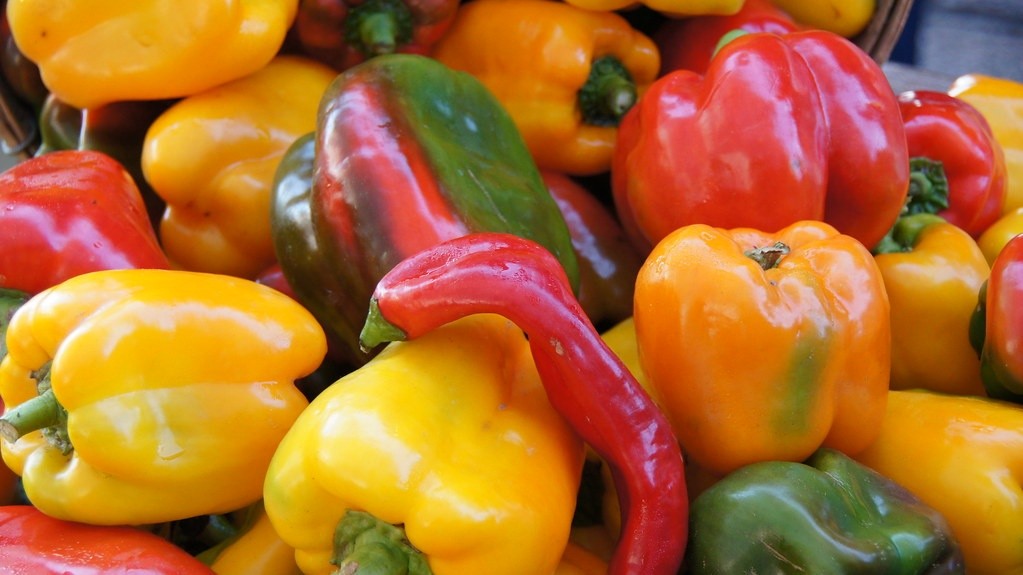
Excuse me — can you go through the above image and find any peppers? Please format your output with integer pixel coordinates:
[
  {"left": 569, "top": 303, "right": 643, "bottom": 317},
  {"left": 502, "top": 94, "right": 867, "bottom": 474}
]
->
[{"left": 0, "top": 2, "right": 1018, "bottom": 574}]
